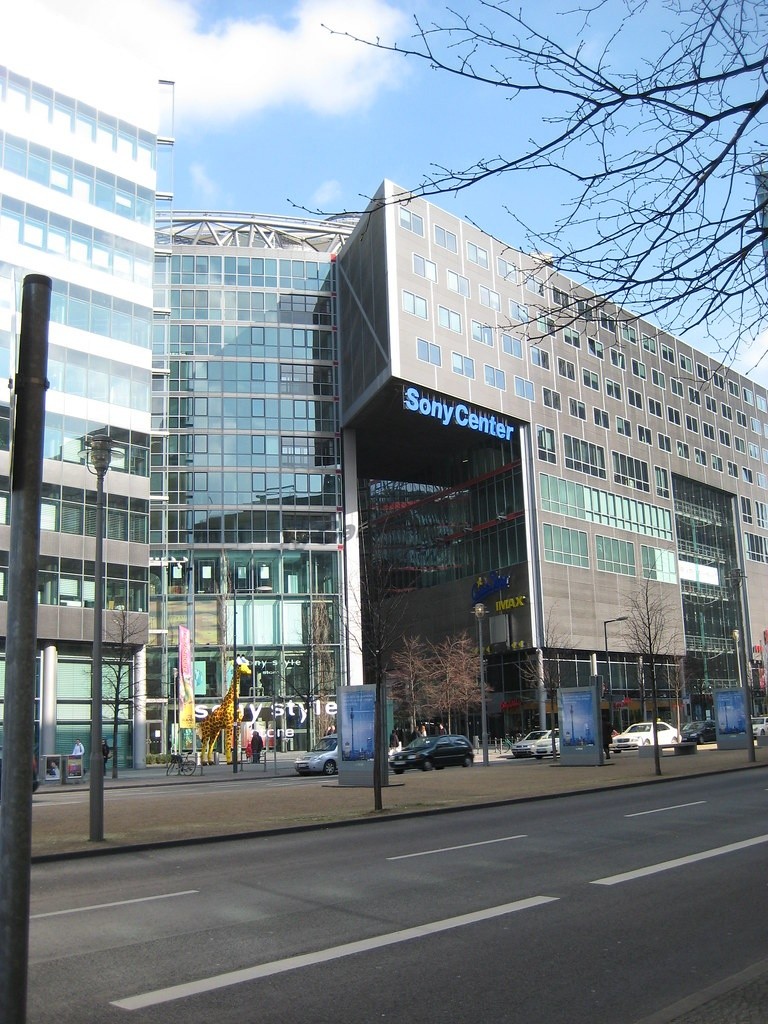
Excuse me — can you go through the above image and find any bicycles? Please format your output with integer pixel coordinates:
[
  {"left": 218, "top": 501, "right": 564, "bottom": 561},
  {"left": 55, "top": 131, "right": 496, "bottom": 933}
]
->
[{"left": 166, "top": 750, "right": 196, "bottom": 776}]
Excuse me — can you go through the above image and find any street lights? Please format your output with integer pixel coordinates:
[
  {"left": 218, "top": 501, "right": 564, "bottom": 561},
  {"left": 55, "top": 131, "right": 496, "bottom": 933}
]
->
[
  {"left": 472, "top": 603, "right": 495, "bottom": 766},
  {"left": 233, "top": 586, "right": 274, "bottom": 770},
  {"left": 79, "top": 433, "right": 123, "bottom": 844},
  {"left": 733, "top": 629, "right": 743, "bottom": 687},
  {"left": 603, "top": 616, "right": 630, "bottom": 729},
  {"left": 725, "top": 567, "right": 759, "bottom": 762}
]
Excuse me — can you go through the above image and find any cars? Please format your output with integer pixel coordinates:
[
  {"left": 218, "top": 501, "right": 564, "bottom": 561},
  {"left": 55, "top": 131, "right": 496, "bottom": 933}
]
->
[
  {"left": 388, "top": 733, "right": 476, "bottom": 775},
  {"left": 511, "top": 728, "right": 561, "bottom": 758},
  {"left": 751, "top": 717, "right": 768, "bottom": 738},
  {"left": 680, "top": 720, "right": 717, "bottom": 745},
  {"left": 295, "top": 734, "right": 338, "bottom": 776},
  {"left": 611, "top": 721, "right": 683, "bottom": 753}
]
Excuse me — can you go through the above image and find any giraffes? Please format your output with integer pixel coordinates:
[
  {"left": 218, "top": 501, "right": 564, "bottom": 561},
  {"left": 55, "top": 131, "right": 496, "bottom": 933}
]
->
[{"left": 200, "top": 662, "right": 253, "bottom": 767}]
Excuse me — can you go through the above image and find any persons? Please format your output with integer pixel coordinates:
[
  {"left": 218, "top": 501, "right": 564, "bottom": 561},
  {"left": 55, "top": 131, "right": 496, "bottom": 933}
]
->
[
  {"left": 102, "top": 739, "right": 109, "bottom": 776},
  {"left": 411, "top": 726, "right": 426, "bottom": 741},
  {"left": 246, "top": 731, "right": 263, "bottom": 764},
  {"left": 390, "top": 729, "right": 400, "bottom": 756},
  {"left": 439, "top": 723, "right": 446, "bottom": 734},
  {"left": 73, "top": 739, "right": 87, "bottom": 775},
  {"left": 602, "top": 716, "right": 613, "bottom": 759},
  {"left": 50, "top": 761, "right": 60, "bottom": 778}
]
